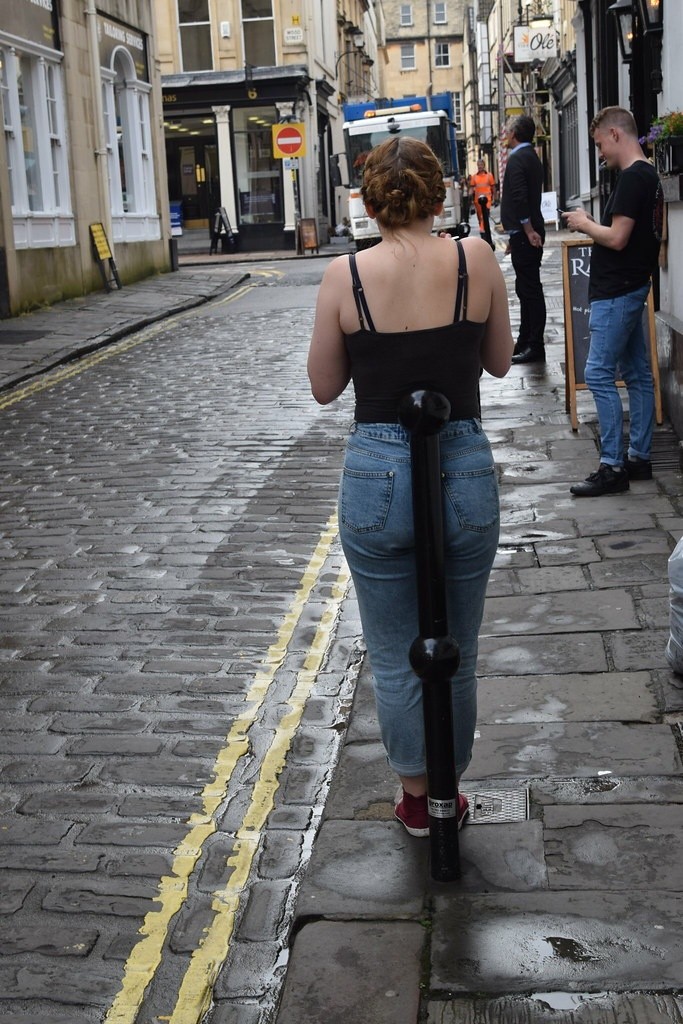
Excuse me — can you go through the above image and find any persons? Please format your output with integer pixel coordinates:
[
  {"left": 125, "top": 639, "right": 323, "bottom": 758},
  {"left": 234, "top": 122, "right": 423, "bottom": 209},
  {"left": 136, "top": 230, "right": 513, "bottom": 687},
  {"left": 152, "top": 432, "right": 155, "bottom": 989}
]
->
[
  {"left": 469, "top": 159, "right": 496, "bottom": 238},
  {"left": 308, "top": 136, "right": 515, "bottom": 838},
  {"left": 459, "top": 175, "right": 474, "bottom": 222},
  {"left": 500, "top": 115, "right": 546, "bottom": 362},
  {"left": 561, "top": 106, "right": 663, "bottom": 498}
]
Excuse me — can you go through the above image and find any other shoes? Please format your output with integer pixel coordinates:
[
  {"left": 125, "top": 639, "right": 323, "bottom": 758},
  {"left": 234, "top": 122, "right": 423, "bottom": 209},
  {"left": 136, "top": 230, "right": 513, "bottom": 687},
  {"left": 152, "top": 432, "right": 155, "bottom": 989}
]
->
[
  {"left": 512, "top": 343, "right": 528, "bottom": 356},
  {"left": 394, "top": 786, "right": 430, "bottom": 836},
  {"left": 511, "top": 347, "right": 546, "bottom": 363},
  {"left": 458, "top": 793, "right": 469, "bottom": 830}
]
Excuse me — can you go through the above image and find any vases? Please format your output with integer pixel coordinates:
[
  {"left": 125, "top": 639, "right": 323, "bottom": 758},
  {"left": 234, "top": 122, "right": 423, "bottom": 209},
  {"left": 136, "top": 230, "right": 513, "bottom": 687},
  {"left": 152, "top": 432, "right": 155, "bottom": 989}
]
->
[{"left": 655, "top": 138, "right": 683, "bottom": 176}]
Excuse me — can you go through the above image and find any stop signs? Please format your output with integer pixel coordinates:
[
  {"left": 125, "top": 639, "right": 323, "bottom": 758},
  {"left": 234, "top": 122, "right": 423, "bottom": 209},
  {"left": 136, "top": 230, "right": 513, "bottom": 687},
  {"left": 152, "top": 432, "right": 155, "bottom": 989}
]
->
[{"left": 272, "top": 123, "right": 307, "bottom": 159}]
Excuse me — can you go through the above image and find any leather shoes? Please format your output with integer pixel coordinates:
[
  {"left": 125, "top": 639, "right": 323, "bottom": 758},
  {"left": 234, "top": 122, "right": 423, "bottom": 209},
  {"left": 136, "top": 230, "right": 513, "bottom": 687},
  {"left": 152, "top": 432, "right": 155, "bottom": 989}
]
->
[
  {"left": 590, "top": 452, "right": 652, "bottom": 480},
  {"left": 570, "top": 462, "right": 630, "bottom": 496}
]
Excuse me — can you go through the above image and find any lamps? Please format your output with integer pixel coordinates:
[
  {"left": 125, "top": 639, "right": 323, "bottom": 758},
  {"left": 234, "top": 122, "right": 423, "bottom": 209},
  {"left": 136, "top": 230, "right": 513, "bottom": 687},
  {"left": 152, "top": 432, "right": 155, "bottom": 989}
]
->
[
  {"left": 607, "top": 0, "right": 632, "bottom": 75},
  {"left": 631, "top": 0, "right": 663, "bottom": 94}
]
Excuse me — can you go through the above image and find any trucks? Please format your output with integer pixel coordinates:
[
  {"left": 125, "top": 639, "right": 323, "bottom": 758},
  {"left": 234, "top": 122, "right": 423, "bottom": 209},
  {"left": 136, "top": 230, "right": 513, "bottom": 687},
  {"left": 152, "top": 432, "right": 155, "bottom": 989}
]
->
[{"left": 342, "top": 104, "right": 472, "bottom": 251}]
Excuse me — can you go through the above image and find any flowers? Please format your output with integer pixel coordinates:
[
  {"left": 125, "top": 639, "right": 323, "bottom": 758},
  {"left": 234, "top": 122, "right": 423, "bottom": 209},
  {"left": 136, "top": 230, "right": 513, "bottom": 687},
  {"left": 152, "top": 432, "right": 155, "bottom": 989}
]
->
[{"left": 638, "top": 111, "right": 683, "bottom": 145}]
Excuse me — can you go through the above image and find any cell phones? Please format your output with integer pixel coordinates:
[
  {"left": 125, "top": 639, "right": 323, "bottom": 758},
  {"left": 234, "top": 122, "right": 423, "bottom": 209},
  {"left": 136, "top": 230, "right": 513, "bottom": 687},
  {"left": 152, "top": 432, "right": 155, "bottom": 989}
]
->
[{"left": 557, "top": 208, "right": 564, "bottom": 213}]
